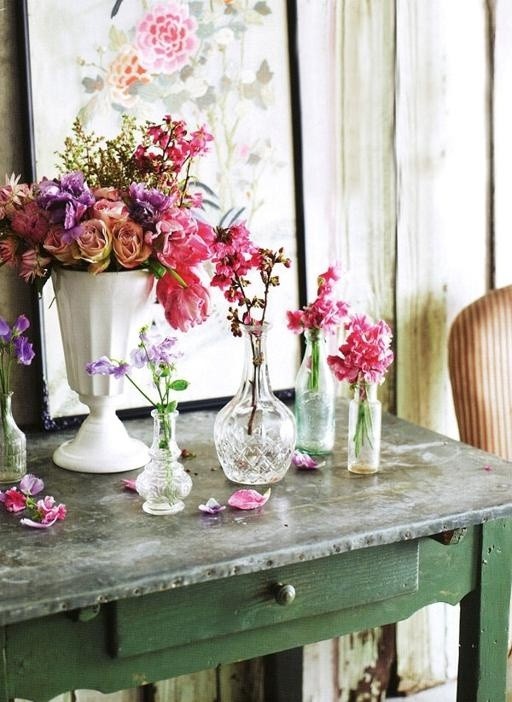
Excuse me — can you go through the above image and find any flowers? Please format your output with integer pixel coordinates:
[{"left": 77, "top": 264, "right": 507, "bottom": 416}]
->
[
  {"left": 327, "top": 316, "right": 394, "bottom": 456},
  {"left": 85, "top": 321, "right": 200, "bottom": 504},
  {"left": 212, "top": 223, "right": 292, "bottom": 434},
  {"left": 2, "top": 113, "right": 255, "bottom": 333},
  {"left": 0, "top": 313, "right": 35, "bottom": 481},
  {"left": 287, "top": 262, "right": 350, "bottom": 390}
]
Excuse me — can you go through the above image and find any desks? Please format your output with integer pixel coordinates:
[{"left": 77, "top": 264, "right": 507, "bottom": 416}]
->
[{"left": 0, "top": 401, "right": 512, "bottom": 701}]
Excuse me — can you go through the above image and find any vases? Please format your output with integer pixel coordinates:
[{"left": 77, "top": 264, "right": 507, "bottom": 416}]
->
[
  {"left": 136, "top": 409, "right": 194, "bottom": 518},
  {"left": 296, "top": 327, "right": 335, "bottom": 454},
  {"left": 1, "top": 390, "right": 27, "bottom": 483},
  {"left": 44, "top": 262, "right": 164, "bottom": 475},
  {"left": 213, "top": 323, "right": 297, "bottom": 491},
  {"left": 347, "top": 379, "right": 381, "bottom": 477}
]
